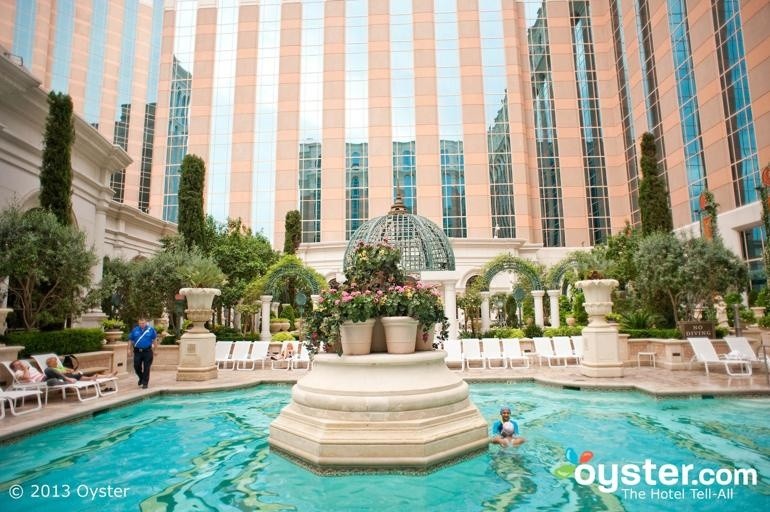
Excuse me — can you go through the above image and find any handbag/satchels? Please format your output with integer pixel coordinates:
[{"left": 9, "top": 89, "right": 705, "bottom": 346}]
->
[{"left": 62, "top": 355, "right": 79, "bottom": 370}]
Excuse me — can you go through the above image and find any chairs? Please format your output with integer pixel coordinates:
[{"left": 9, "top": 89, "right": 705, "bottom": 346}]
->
[
  {"left": 686, "top": 336, "right": 770, "bottom": 377},
  {"left": 0, "top": 352, "right": 119, "bottom": 416},
  {"left": 443, "top": 335, "right": 583, "bottom": 370},
  {"left": 216, "top": 340, "right": 324, "bottom": 370}
]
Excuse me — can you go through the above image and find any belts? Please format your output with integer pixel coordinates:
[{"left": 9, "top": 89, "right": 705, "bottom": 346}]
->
[{"left": 134, "top": 348, "right": 150, "bottom": 352}]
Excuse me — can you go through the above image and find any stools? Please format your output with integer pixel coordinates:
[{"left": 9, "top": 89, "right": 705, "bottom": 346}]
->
[{"left": 638, "top": 351, "right": 657, "bottom": 368}]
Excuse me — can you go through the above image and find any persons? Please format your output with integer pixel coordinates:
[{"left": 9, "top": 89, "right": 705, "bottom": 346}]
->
[
  {"left": 126, "top": 315, "right": 159, "bottom": 390},
  {"left": 488, "top": 419, "right": 527, "bottom": 451},
  {"left": 713, "top": 294, "right": 731, "bottom": 328},
  {"left": 45, "top": 356, "right": 117, "bottom": 382},
  {"left": 491, "top": 405, "right": 521, "bottom": 438},
  {"left": 8, "top": 359, "right": 77, "bottom": 387}
]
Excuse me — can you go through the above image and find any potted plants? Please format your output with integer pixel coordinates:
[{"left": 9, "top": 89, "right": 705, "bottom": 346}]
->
[
  {"left": 98, "top": 319, "right": 125, "bottom": 345},
  {"left": 564, "top": 264, "right": 623, "bottom": 327},
  {"left": 177, "top": 265, "right": 223, "bottom": 334},
  {"left": 270, "top": 318, "right": 304, "bottom": 333}
]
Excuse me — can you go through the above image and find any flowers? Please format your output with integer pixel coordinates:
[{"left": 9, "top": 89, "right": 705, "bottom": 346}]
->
[{"left": 303, "top": 235, "right": 448, "bottom": 359}]
